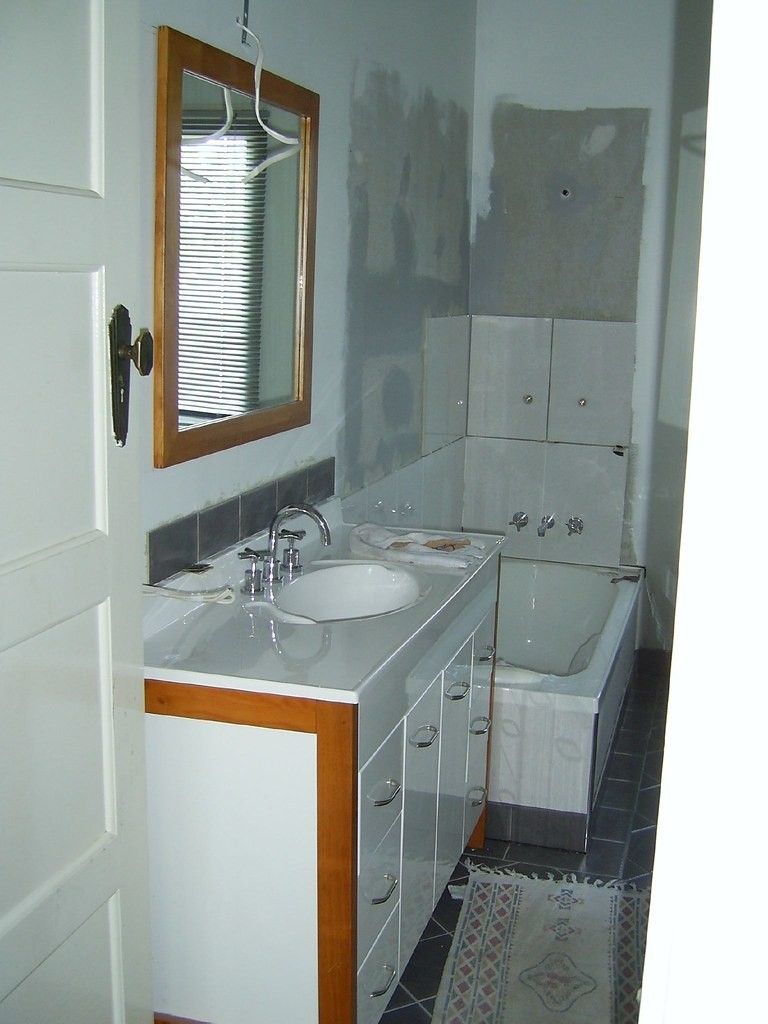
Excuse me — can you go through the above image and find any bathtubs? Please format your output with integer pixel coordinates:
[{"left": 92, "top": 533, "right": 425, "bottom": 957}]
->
[{"left": 485, "top": 555, "right": 646, "bottom": 852}]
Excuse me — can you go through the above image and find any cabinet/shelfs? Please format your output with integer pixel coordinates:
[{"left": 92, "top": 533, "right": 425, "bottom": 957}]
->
[{"left": 141, "top": 550, "right": 501, "bottom": 1024}]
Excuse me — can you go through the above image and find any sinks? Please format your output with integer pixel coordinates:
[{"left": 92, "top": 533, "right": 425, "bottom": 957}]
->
[{"left": 275, "top": 560, "right": 432, "bottom": 624}]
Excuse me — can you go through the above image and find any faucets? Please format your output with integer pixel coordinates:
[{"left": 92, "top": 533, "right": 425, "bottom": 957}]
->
[
  {"left": 268, "top": 620, "right": 332, "bottom": 669},
  {"left": 537, "top": 515, "right": 555, "bottom": 537},
  {"left": 261, "top": 502, "right": 331, "bottom": 584}
]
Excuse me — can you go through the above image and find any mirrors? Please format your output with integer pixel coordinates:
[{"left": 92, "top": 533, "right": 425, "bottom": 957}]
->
[{"left": 152, "top": 25, "right": 311, "bottom": 470}]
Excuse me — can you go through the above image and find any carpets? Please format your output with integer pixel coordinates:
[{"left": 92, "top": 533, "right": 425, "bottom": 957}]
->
[{"left": 429, "top": 859, "right": 652, "bottom": 1024}]
